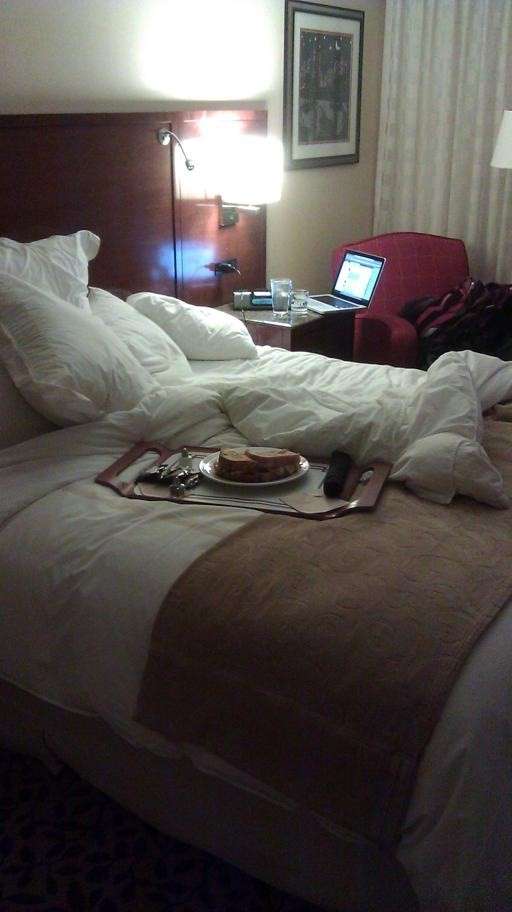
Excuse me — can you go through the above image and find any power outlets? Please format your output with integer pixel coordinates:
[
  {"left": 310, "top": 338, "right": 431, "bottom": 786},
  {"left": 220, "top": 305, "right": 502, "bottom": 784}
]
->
[{"left": 215, "top": 259, "right": 238, "bottom": 276}]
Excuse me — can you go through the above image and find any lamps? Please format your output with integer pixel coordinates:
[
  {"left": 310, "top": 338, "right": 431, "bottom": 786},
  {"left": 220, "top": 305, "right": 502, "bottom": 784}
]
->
[
  {"left": 490, "top": 108, "right": 512, "bottom": 169},
  {"left": 220, "top": 133, "right": 284, "bottom": 227}
]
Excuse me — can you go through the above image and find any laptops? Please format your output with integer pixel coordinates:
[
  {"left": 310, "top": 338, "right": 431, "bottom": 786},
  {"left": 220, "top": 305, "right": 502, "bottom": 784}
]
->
[{"left": 307, "top": 249, "right": 386, "bottom": 315}]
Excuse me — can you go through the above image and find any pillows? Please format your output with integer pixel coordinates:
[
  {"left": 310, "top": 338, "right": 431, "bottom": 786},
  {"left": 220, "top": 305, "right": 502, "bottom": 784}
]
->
[
  {"left": 0, "top": 230, "right": 101, "bottom": 311},
  {"left": 88, "top": 285, "right": 193, "bottom": 386},
  {"left": 126, "top": 291, "right": 260, "bottom": 360},
  {"left": 0, "top": 363, "right": 63, "bottom": 450},
  {"left": 0, "top": 269, "right": 161, "bottom": 428}
]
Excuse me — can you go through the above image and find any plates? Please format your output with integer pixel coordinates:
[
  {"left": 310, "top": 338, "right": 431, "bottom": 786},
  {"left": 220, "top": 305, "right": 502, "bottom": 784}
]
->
[{"left": 198, "top": 445, "right": 310, "bottom": 486}]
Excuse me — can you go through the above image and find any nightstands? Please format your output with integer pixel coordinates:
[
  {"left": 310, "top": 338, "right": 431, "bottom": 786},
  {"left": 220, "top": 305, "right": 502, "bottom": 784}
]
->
[{"left": 213, "top": 301, "right": 355, "bottom": 362}]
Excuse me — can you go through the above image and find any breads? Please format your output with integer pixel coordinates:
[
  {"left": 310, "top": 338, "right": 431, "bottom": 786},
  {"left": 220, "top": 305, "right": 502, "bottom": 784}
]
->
[{"left": 212, "top": 447, "right": 301, "bottom": 483}]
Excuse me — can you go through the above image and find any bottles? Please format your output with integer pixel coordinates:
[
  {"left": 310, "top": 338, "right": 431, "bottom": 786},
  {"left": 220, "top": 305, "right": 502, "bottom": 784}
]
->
[{"left": 177, "top": 448, "right": 194, "bottom": 471}]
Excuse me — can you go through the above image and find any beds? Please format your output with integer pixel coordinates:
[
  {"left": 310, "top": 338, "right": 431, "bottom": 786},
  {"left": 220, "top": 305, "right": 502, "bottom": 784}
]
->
[{"left": 0, "top": 110, "right": 512, "bottom": 912}]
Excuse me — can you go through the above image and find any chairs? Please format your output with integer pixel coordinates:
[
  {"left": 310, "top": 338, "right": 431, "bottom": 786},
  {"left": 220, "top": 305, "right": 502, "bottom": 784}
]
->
[{"left": 332, "top": 232, "right": 469, "bottom": 368}]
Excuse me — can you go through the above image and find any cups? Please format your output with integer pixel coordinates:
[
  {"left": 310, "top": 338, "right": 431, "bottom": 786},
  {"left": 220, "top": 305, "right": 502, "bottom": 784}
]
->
[
  {"left": 269, "top": 277, "right": 292, "bottom": 317},
  {"left": 290, "top": 289, "right": 309, "bottom": 312}
]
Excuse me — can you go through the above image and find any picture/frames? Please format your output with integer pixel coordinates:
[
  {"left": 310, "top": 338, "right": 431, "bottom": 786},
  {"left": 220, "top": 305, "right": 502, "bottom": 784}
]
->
[{"left": 282, "top": 0, "right": 365, "bottom": 171}]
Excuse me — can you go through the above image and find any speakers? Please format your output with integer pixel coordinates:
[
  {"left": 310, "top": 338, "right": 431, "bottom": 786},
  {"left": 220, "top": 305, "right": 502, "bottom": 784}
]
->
[{"left": 233, "top": 288, "right": 273, "bottom": 311}]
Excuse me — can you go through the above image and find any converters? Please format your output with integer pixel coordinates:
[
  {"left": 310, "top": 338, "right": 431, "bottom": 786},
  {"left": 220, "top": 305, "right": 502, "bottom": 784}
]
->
[{"left": 221, "top": 263, "right": 232, "bottom": 273}]
